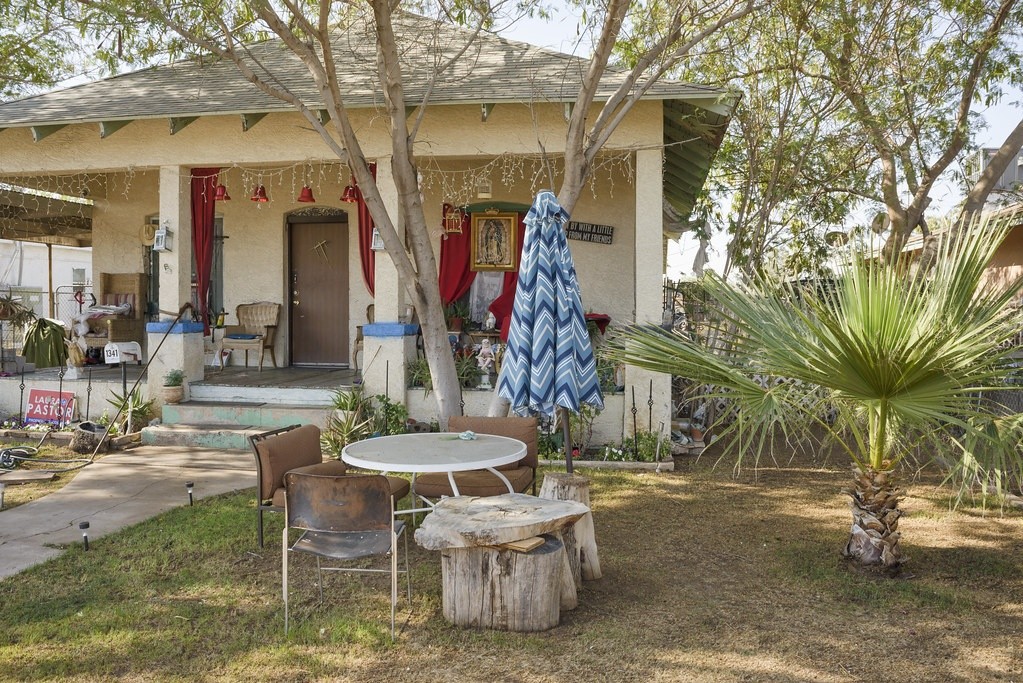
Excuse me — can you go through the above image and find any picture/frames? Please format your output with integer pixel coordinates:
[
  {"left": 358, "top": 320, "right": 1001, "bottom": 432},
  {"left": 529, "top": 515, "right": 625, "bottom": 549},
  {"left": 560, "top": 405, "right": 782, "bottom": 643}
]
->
[{"left": 470, "top": 212, "right": 519, "bottom": 272}]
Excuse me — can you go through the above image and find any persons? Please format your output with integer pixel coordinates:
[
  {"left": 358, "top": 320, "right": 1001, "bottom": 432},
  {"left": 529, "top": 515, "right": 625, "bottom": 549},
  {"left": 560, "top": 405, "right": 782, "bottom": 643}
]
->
[{"left": 475, "top": 338, "right": 495, "bottom": 371}]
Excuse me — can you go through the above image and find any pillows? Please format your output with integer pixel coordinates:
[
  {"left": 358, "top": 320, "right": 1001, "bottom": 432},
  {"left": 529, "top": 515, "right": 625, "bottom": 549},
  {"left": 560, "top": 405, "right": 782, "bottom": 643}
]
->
[{"left": 283, "top": 460, "right": 346, "bottom": 490}]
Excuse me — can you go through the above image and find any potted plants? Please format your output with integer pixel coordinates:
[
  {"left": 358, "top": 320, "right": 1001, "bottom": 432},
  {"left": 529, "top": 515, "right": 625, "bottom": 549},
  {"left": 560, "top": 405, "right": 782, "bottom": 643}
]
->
[
  {"left": 447, "top": 299, "right": 471, "bottom": 332},
  {"left": 331, "top": 390, "right": 375, "bottom": 428},
  {"left": 161, "top": 368, "right": 187, "bottom": 404}
]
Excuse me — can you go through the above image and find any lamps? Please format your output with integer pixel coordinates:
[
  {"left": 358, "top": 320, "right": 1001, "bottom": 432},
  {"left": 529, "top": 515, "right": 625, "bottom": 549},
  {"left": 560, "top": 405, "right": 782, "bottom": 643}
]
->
[
  {"left": 369, "top": 227, "right": 385, "bottom": 252},
  {"left": 152, "top": 220, "right": 173, "bottom": 253}
]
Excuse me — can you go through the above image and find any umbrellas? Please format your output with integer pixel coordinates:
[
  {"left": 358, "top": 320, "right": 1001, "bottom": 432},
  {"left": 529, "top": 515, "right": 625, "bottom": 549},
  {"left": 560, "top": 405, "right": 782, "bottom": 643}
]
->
[{"left": 494, "top": 187, "right": 604, "bottom": 473}]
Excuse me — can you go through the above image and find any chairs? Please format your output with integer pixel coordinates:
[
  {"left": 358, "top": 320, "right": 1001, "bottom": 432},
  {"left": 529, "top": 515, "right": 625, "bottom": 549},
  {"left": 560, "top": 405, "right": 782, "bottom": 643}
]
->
[
  {"left": 353, "top": 303, "right": 415, "bottom": 374},
  {"left": 281, "top": 472, "right": 415, "bottom": 644},
  {"left": 248, "top": 422, "right": 409, "bottom": 549},
  {"left": 218, "top": 300, "right": 280, "bottom": 372},
  {"left": 70, "top": 272, "right": 150, "bottom": 366},
  {"left": 413, "top": 415, "right": 539, "bottom": 520}
]
